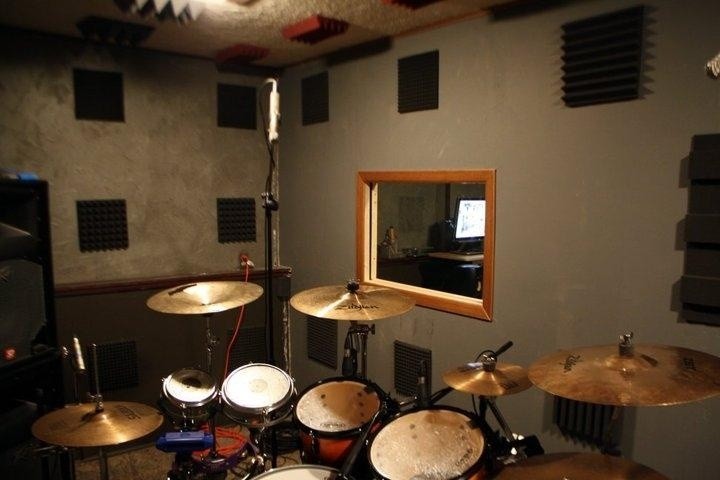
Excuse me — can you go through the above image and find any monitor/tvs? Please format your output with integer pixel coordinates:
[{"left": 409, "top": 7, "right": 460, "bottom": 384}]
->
[{"left": 452, "top": 196, "right": 486, "bottom": 255}]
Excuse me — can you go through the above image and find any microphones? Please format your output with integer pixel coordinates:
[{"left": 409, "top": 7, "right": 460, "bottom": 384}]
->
[
  {"left": 267, "top": 82, "right": 281, "bottom": 145},
  {"left": 488, "top": 339, "right": 514, "bottom": 358}
]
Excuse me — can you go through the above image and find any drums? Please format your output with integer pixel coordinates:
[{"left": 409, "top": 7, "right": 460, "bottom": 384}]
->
[
  {"left": 157, "top": 366, "right": 219, "bottom": 424},
  {"left": 220, "top": 362, "right": 298, "bottom": 426},
  {"left": 244, "top": 464, "right": 355, "bottom": 480},
  {"left": 293, "top": 376, "right": 397, "bottom": 475},
  {"left": 365, "top": 405, "right": 490, "bottom": 480}
]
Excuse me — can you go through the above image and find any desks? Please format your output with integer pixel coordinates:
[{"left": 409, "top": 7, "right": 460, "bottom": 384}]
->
[{"left": 425, "top": 251, "right": 484, "bottom": 298}]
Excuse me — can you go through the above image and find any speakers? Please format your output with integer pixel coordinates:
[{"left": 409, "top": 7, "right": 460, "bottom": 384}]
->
[
  {"left": 0, "top": 349, "right": 76, "bottom": 480},
  {"left": 0, "top": 173, "right": 66, "bottom": 369}
]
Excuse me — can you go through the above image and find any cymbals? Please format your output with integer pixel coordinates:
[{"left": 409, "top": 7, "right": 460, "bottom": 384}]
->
[
  {"left": 494, "top": 451, "right": 671, "bottom": 480},
  {"left": 442, "top": 362, "right": 533, "bottom": 396},
  {"left": 291, "top": 284, "right": 415, "bottom": 321},
  {"left": 528, "top": 344, "right": 720, "bottom": 406},
  {"left": 147, "top": 280, "right": 264, "bottom": 315},
  {"left": 31, "top": 400, "right": 163, "bottom": 446}
]
